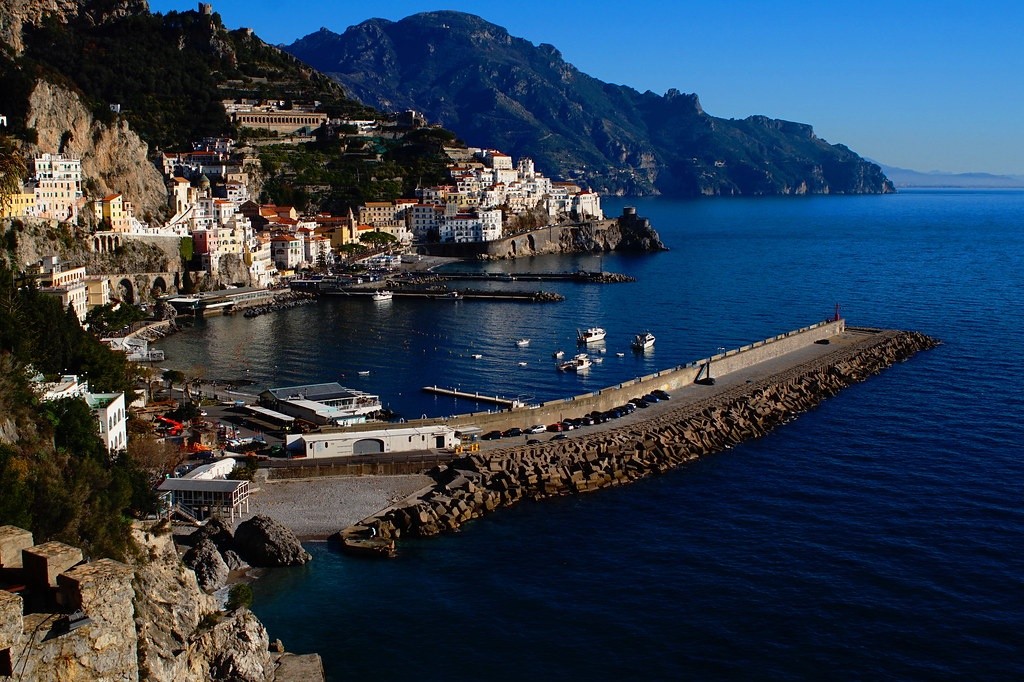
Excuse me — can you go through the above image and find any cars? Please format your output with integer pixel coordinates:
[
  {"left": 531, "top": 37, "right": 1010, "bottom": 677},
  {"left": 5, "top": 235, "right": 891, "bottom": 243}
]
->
[
  {"left": 642, "top": 393, "right": 659, "bottom": 403},
  {"left": 650, "top": 389, "right": 671, "bottom": 400},
  {"left": 549, "top": 434, "right": 567, "bottom": 442},
  {"left": 547, "top": 424, "right": 563, "bottom": 431},
  {"left": 526, "top": 439, "right": 542, "bottom": 444},
  {"left": 526, "top": 424, "right": 546, "bottom": 434},
  {"left": 482, "top": 430, "right": 503, "bottom": 441},
  {"left": 561, "top": 422, "right": 574, "bottom": 431},
  {"left": 585, "top": 402, "right": 636, "bottom": 424},
  {"left": 816, "top": 339, "right": 830, "bottom": 344},
  {"left": 503, "top": 427, "right": 523, "bottom": 438},
  {"left": 575, "top": 418, "right": 586, "bottom": 425},
  {"left": 583, "top": 417, "right": 594, "bottom": 425},
  {"left": 563, "top": 418, "right": 581, "bottom": 429},
  {"left": 628, "top": 398, "right": 650, "bottom": 408}
]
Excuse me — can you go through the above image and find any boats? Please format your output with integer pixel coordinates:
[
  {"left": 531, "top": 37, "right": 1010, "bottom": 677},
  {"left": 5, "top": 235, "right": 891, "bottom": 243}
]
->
[
  {"left": 599, "top": 348, "right": 607, "bottom": 352},
  {"left": 372, "top": 290, "right": 393, "bottom": 301},
  {"left": 572, "top": 354, "right": 589, "bottom": 359},
  {"left": 569, "top": 359, "right": 592, "bottom": 370},
  {"left": 617, "top": 353, "right": 625, "bottom": 356},
  {"left": 594, "top": 358, "right": 603, "bottom": 363},
  {"left": 516, "top": 339, "right": 530, "bottom": 346},
  {"left": 554, "top": 348, "right": 565, "bottom": 357},
  {"left": 555, "top": 361, "right": 578, "bottom": 370},
  {"left": 631, "top": 332, "right": 655, "bottom": 349},
  {"left": 576, "top": 327, "right": 607, "bottom": 343}
]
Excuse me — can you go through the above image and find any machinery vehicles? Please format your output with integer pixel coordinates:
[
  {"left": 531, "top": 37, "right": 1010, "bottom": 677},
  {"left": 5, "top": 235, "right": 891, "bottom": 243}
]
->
[{"left": 157, "top": 414, "right": 185, "bottom": 436}]
